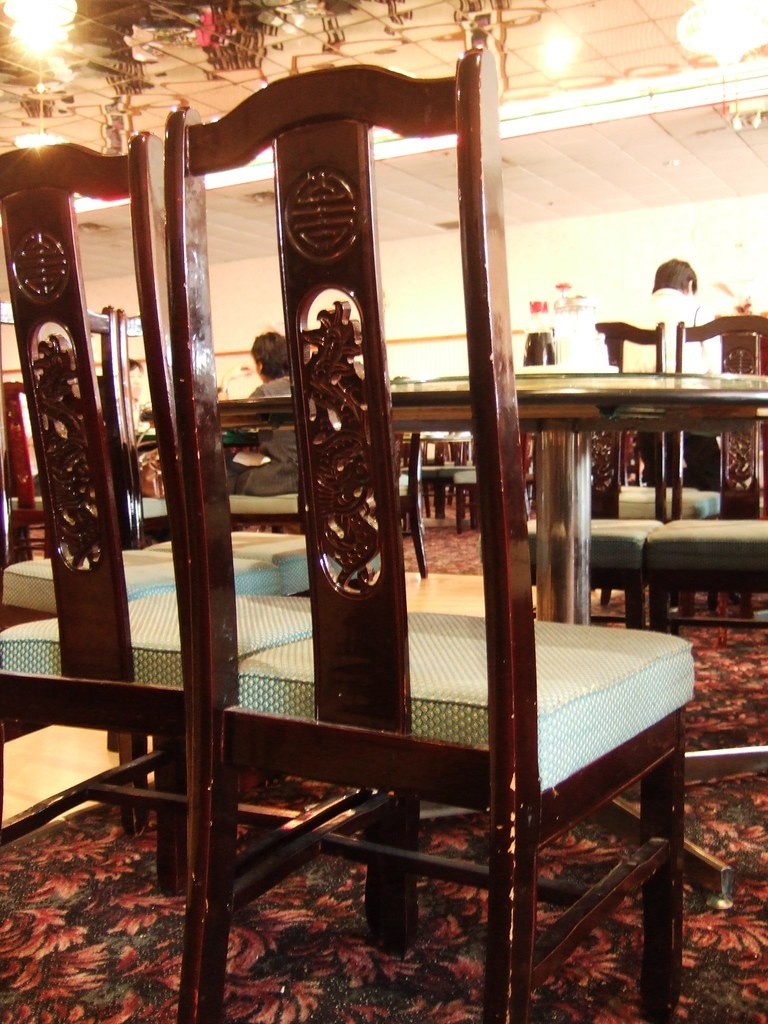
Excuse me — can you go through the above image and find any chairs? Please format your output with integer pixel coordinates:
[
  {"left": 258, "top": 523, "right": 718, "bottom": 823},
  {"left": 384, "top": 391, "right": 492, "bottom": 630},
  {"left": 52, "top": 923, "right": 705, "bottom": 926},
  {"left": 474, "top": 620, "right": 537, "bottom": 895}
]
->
[{"left": 1, "top": 45, "right": 768, "bottom": 1024}]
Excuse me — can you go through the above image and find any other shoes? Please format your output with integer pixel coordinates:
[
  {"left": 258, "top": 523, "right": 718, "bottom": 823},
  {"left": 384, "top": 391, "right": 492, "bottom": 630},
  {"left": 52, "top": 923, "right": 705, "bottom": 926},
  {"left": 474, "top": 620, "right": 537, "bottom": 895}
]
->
[{"left": 729, "top": 592, "right": 740, "bottom": 603}]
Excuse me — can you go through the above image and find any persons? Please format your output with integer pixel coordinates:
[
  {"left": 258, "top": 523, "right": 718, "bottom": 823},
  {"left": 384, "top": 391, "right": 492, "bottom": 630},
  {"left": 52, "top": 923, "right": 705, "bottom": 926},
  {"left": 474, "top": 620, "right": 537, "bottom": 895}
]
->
[
  {"left": 227, "top": 331, "right": 301, "bottom": 495},
  {"left": 126, "top": 359, "right": 146, "bottom": 436},
  {"left": 614, "top": 259, "right": 723, "bottom": 492}
]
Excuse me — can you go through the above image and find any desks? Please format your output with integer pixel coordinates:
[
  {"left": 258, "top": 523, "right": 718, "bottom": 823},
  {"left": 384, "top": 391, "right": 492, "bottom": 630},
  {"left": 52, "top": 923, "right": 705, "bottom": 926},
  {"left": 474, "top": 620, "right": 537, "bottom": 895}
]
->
[{"left": 221, "top": 372, "right": 768, "bottom": 910}]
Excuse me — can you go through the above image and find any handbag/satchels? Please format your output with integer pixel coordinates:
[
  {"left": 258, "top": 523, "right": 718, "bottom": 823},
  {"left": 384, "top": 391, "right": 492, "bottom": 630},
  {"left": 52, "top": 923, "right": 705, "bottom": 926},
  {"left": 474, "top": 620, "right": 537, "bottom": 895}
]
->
[{"left": 135, "top": 427, "right": 165, "bottom": 499}]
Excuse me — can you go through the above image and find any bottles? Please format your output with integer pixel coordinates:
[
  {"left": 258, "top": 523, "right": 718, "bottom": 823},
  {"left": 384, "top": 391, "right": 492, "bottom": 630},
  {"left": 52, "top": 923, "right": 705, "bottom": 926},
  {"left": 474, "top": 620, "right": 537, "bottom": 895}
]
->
[
  {"left": 553, "top": 298, "right": 597, "bottom": 369},
  {"left": 523, "top": 301, "right": 555, "bottom": 367}
]
